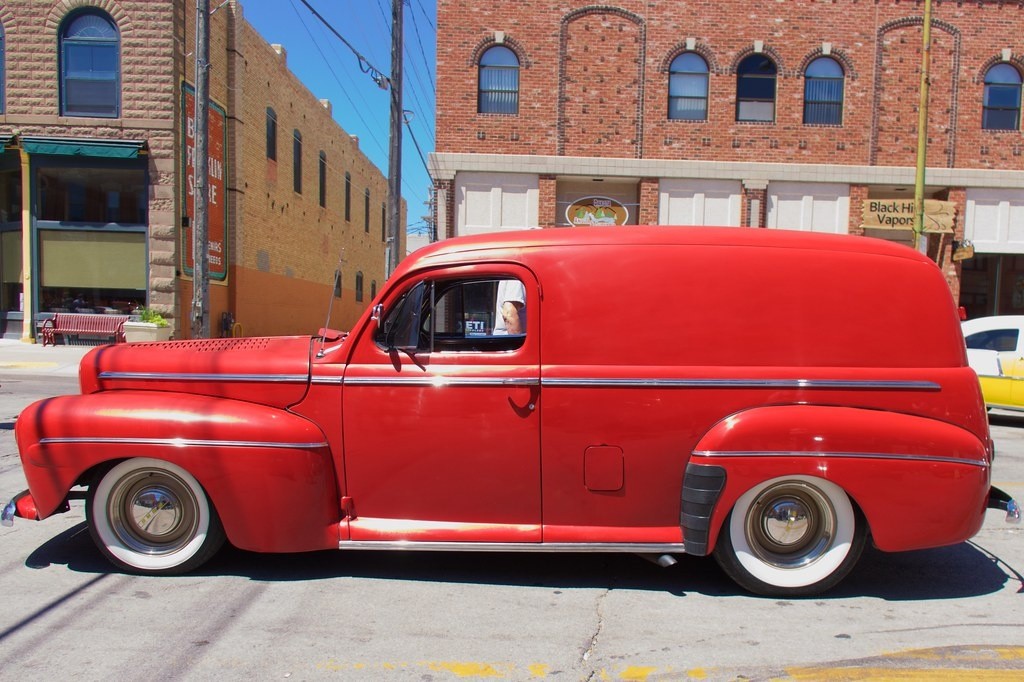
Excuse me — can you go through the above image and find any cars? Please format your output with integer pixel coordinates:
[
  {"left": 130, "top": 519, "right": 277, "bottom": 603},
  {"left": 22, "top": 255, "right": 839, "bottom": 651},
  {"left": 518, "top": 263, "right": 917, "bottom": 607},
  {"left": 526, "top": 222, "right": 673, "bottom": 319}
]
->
[
  {"left": 0, "top": 224, "right": 1024, "bottom": 599},
  {"left": 960, "top": 314, "right": 1023, "bottom": 422}
]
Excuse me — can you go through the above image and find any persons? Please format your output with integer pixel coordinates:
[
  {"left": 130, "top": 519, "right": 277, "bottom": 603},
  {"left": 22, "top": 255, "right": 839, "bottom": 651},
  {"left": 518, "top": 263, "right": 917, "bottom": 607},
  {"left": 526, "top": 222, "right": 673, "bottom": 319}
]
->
[{"left": 493, "top": 280, "right": 526, "bottom": 334}]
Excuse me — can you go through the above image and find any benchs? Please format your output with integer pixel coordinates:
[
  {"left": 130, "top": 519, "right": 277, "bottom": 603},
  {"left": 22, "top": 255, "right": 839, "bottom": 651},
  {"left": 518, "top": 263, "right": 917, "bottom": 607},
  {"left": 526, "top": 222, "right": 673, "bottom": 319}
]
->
[{"left": 42, "top": 313, "right": 130, "bottom": 347}]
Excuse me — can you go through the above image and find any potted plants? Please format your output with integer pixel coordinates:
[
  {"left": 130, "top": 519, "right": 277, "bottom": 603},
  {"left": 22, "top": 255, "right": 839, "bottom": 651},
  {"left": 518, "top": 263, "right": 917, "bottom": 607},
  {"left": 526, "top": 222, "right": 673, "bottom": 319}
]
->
[{"left": 122, "top": 305, "right": 173, "bottom": 342}]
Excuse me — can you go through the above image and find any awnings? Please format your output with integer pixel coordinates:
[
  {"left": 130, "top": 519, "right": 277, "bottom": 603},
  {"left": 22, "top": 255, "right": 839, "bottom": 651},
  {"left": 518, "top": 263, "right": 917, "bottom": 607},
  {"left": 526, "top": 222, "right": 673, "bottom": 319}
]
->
[{"left": 19, "top": 135, "right": 148, "bottom": 160}]
